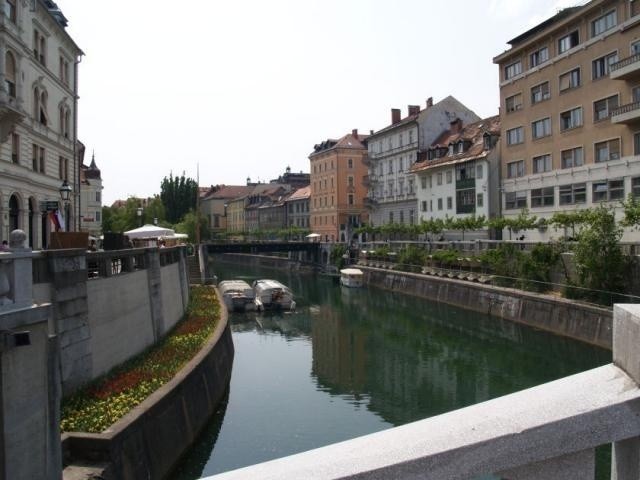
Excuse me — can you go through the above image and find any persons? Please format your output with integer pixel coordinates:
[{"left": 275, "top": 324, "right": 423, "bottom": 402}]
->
[
  {"left": 89, "top": 239, "right": 96, "bottom": 251},
  {"left": 0, "top": 240, "right": 9, "bottom": 250}
]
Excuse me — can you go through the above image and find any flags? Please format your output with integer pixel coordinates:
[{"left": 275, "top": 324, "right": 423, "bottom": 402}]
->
[{"left": 48, "top": 210, "right": 66, "bottom": 232}]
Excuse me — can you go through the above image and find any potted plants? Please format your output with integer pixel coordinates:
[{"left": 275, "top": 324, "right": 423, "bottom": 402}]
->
[{"left": 355, "top": 246, "right": 497, "bottom": 283}]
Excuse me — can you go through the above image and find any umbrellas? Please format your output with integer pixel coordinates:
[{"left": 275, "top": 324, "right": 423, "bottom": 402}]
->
[
  {"left": 159, "top": 232, "right": 189, "bottom": 240},
  {"left": 121, "top": 223, "right": 175, "bottom": 237},
  {"left": 306, "top": 233, "right": 321, "bottom": 238}
]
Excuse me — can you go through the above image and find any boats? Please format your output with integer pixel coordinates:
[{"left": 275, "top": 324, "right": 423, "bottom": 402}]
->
[
  {"left": 251, "top": 279, "right": 297, "bottom": 311},
  {"left": 339, "top": 268, "right": 364, "bottom": 287},
  {"left": 217, "top": 279, "right": 255, "bottom": 313}
]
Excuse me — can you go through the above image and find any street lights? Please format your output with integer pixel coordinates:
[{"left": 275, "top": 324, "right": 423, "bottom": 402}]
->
[
  {"left": 153, "top": 217, "right": 158, "bottom": 226},
  {"left": 60, "top": 176, "right": 73, "bottom": 232},
  {"left": 136, "top": 207, "right": 144, "bottom": 228}
]
